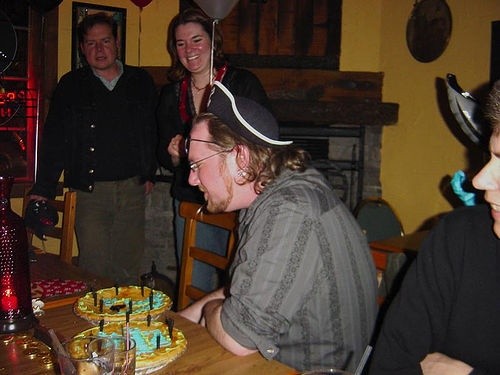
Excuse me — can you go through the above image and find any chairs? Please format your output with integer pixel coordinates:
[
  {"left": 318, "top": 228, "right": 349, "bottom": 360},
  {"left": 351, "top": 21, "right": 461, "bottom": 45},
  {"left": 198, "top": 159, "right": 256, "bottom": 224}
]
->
[
  {"left": 176, "top": 202, "right": 239, "bottom": 314},
  {"left": 20, "top": 186, "right": 78, "bottom": 266}
]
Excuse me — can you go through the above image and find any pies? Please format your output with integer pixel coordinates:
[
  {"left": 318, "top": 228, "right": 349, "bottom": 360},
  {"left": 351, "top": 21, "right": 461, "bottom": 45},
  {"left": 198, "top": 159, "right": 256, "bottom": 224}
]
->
[
  {"left": 73, "top": 285, "right": 173, "bottom": 321},
  {"left": 67, "top": 320, "right": 187, "bottom": 370}
]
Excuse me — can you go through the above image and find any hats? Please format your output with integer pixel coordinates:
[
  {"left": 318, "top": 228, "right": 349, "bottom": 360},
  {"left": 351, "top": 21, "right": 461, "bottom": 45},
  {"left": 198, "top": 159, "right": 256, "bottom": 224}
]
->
[{"left": 206, "top": 81, "right": 293, "bottom": 148}]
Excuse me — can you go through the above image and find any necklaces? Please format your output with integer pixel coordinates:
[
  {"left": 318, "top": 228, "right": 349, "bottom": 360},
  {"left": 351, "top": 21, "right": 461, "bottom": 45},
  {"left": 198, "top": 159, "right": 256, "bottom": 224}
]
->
[{"left": 191, "top": 76, "right": 215, "bottom": 94}]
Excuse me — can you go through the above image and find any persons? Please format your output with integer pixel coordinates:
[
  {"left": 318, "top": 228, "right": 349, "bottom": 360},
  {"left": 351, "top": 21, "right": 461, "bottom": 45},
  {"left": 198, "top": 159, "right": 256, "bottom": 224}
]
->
[
  {"left": 189, "top": 81, "right": 378, "bottom": 375},
  {"left": 369, "top": 80, "right": 500, "bottom": 375},
  {"left": 30, "top": 11, "right": 161, "bottom": 287},
  {"left": 157, "top": 7, "right": 271, "bottom": 305}
]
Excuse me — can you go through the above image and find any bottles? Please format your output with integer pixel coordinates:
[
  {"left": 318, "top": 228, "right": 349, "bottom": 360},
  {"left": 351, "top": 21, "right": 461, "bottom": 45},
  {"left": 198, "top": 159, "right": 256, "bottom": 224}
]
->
[{"left": 0, "top": 153, "right": 33, "bottom": 335}]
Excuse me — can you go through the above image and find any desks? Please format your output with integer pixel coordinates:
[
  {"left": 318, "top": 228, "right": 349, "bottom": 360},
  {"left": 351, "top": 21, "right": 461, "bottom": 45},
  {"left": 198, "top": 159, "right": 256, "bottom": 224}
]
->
[{"left": 0, "top": 261, "right": 301, "bottom": 375}]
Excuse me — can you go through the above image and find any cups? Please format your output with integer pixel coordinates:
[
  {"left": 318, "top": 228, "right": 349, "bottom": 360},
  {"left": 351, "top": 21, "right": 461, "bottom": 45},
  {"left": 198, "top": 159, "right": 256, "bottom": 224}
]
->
[
  {"left": 55, "top": 336, "right": 115, "bottom": 375},
  {"left": 88, "top": 335, "right": 137, "bottom": 375}
]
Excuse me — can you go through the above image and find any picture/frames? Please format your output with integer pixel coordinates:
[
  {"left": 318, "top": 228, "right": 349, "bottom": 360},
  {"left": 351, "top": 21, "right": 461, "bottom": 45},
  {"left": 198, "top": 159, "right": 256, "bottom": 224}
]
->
[{"left": 70, "top": 1, "right": 127, "bottom": 71}]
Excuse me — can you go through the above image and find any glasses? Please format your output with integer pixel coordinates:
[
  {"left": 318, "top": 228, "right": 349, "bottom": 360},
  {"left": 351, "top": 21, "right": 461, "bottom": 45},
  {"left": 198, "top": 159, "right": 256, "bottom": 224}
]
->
[
  {"left": 185, "top": 136, "right": 223, "bottom": 156},
  {"left": 187, "top": 150, "right": 224, "bottom": 172}
]
